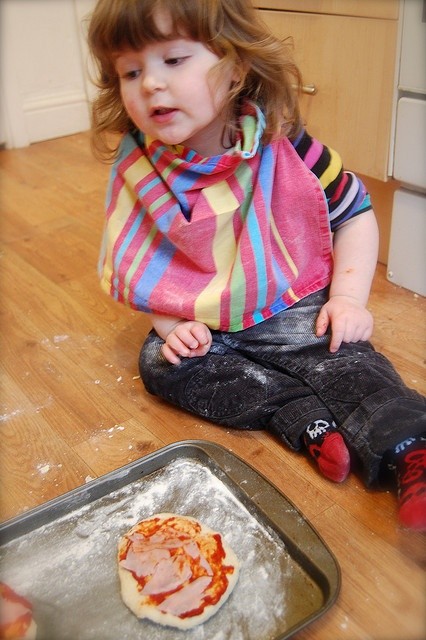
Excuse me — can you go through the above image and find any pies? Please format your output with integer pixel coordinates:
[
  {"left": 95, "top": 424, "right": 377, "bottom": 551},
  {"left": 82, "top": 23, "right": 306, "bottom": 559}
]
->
[{"left": 116, "top": 514, "right": 239, "bottom": 630}]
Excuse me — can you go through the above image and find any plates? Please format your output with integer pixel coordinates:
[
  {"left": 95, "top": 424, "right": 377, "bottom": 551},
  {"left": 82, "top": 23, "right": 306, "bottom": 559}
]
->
[{"left": 0, "top": 439, "right": 341, "bottom": 636}]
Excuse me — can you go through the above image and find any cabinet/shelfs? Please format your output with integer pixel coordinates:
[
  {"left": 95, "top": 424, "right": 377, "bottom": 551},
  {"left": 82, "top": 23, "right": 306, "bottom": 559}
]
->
[
  {"left": 253, "top": 1, "right": 426, "bottom": 298},
  {"left": 0, "top": 0, "right": 105, "bottom": 151}
]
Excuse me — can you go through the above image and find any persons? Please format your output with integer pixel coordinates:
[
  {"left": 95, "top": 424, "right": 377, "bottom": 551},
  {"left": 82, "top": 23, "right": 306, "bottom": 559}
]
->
[{"left": 80, "top": 1, "right": 426, "bottom": 529}]
